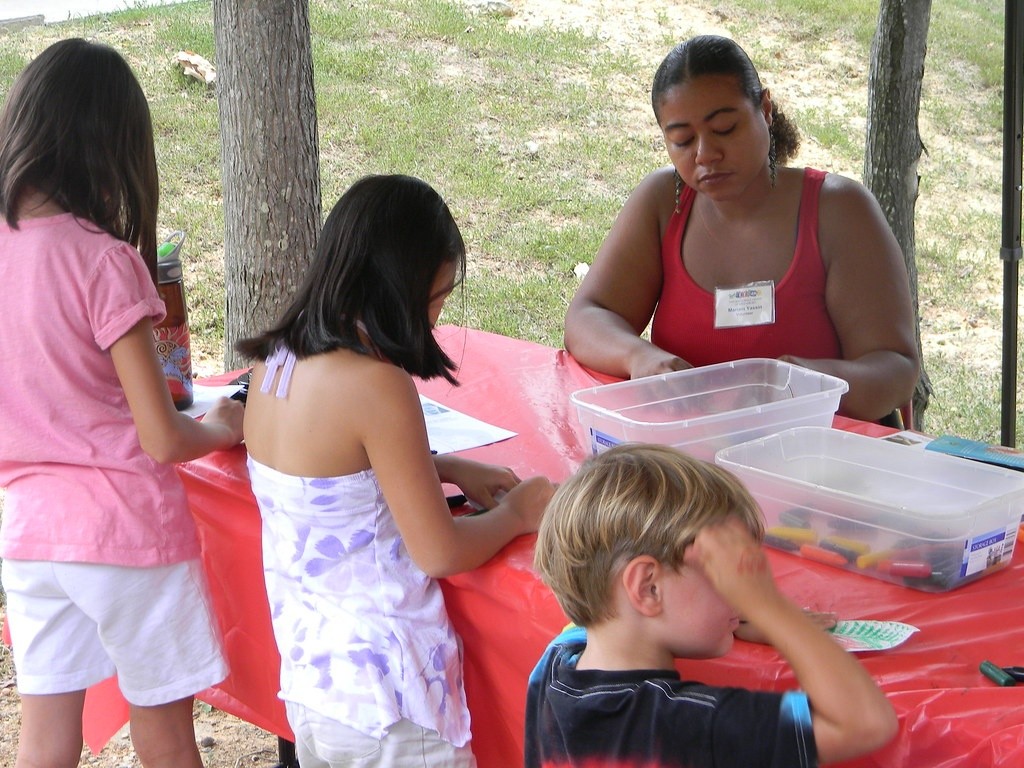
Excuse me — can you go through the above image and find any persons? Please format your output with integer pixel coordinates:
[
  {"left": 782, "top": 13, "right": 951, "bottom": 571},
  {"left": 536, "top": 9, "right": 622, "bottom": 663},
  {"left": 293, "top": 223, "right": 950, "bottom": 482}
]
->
[
  {"left": 521, "top": 445, "right": 897, "bottom": 767},
  {"left": 237, "top": 172, "right": 559, "bottom": 768},
  {"left": 1, "top": 37, "right": 245, "bottom": 768},
  {"left": 563, "top": 35, "right": 918, "bottom": 422}
]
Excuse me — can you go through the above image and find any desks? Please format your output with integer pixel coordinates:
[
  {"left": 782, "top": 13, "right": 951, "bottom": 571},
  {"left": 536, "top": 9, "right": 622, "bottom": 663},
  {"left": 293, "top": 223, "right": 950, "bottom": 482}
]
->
[{"left": 81, "top": 323, "right": 1024, "bottom": 768}]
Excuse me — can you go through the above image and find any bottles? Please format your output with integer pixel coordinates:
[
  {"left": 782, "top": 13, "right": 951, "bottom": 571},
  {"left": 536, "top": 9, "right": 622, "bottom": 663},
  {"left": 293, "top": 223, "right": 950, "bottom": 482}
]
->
[{"left": 152, "top": 231, "right": 193, "bottom": 409}]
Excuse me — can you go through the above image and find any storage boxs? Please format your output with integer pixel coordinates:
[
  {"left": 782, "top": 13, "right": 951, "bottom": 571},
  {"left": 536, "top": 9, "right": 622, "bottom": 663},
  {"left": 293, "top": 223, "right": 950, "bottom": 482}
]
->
[
  {"left": 571, "top": 356, "right": 849, "bottom": 465},
  {"left": 715, "top": 426, "right": 1024, "bottom": 593}
]
